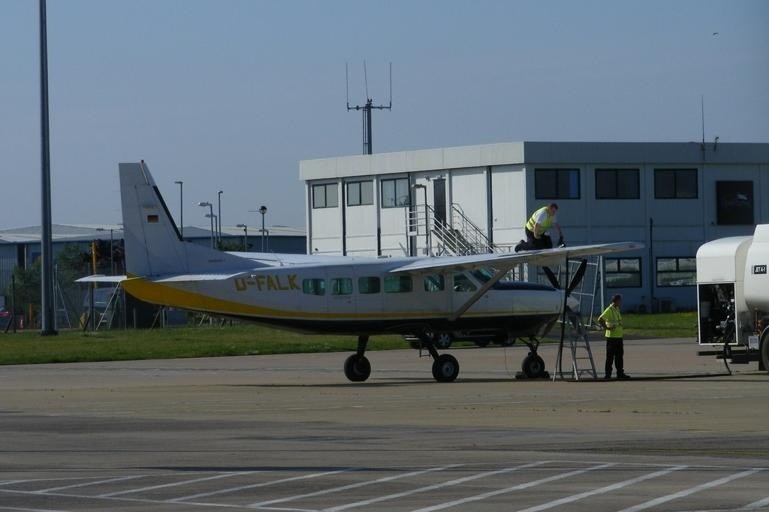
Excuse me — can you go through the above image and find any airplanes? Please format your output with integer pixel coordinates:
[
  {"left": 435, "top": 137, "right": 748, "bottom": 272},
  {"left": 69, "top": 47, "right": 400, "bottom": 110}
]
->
[{"left": 71, "top": 158, "right": 645, "bottom": 383}]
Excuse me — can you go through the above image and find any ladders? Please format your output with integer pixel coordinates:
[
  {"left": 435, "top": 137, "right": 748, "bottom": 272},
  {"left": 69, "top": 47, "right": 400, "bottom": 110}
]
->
[{"left": 552, "top": 312, "right": 597, "bottom": 382}]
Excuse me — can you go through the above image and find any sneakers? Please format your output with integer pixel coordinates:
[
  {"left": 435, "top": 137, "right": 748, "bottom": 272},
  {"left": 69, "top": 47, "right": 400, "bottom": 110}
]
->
[
  {"left": 515, "top": 239, "right": 525, "bottom": 253},
  {"left": 605, "top": 373, "right": 630, "bottom": 379}
]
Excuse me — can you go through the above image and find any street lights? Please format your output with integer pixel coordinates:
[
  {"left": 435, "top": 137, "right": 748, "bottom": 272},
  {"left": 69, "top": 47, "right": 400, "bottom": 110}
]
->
[
  {"left": 95, "top": 227, "right": 123, "bottom": 275},
  {"left": 174, "top": 180, "right": 271, "bottom": 252}
]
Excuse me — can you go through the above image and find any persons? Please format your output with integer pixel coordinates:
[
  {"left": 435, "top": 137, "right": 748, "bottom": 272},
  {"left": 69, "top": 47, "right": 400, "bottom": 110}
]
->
[
  {"left": 515, "top": 203, "right": 563, "bottom": 254},
  {"left": 598, "top": 293, "right": 631, "bottom": 380}
]
